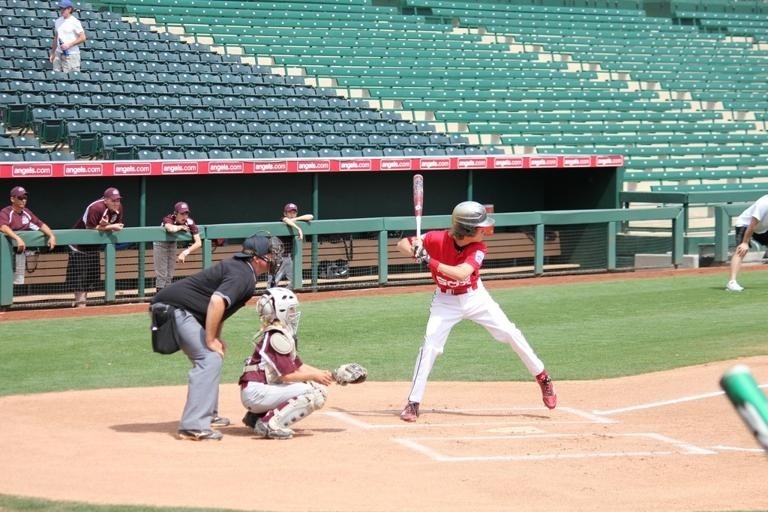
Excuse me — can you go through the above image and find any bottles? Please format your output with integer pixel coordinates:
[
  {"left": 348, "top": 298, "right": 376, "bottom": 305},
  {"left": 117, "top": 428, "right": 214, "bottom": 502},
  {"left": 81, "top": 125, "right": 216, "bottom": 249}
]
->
[{"left": 58, "top": 37, "right": 69, "bottom": 56}]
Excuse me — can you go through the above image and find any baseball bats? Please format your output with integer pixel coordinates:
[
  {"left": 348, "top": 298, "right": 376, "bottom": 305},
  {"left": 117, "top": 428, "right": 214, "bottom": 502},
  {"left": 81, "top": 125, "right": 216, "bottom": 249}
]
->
[{"left": 413, "top": 174, "right": 424, "bottom": 237}]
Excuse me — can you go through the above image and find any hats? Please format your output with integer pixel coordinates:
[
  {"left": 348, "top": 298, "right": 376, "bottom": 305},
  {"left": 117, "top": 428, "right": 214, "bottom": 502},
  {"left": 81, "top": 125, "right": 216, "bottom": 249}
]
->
[
  {"left": 284, "top": 203, "right": 297, "bottom": 212},
  {"left": 10, "top": 187, "right": 28, "bottom": 196},
  {"left": 175, "top": 202, "right": 190, "bottom": 213},
  {"left": 59, "top": 0, "right": 73, "bottom": 9},
  {"left": 104, "top": 187, "right": 122, "bottom": 199}
]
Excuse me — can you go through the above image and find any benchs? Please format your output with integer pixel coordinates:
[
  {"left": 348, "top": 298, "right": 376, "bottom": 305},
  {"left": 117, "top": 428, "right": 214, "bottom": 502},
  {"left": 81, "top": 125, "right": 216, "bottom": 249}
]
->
[
  {"left": 0, "top": 0, "right": 768, "bottom": 171},
  {"left": 625, "top": 169, "right": 768, "bottom": 208}
]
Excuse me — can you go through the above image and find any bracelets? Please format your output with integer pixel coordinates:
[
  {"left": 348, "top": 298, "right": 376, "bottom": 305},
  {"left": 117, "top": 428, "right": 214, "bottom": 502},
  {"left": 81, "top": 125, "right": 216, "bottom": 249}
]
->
[{"left": 182, "top": 250, "right": 189, "bottom": 256}]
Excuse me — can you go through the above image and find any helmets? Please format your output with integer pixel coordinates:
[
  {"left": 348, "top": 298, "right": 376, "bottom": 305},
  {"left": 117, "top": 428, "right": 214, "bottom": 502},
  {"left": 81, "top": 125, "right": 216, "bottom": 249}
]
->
[
  {"left": 256, "top": 287, "right": 300, "bottom": 339},
  {"left": 234, "top": 230, "right": 284, "bottom": 276},
  {"left": 452, "top": 201, "right": 494, "bottom": 240}
]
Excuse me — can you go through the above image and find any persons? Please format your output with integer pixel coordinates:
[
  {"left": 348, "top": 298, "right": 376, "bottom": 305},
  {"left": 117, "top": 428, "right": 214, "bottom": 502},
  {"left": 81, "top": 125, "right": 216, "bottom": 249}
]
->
[
  {"left": 725, "top": 193, "right": 768, "bottom": 292},
  {"left": 0, "top": 185, "right": 56, "bottom": 285},
  {"left": 396, "top": 201, "right": 557, "bottom": 423},
  {"left": 152, "top": 202, "right": 203, "bottom": 293},
  {"left": 50, "top": 0, "right": 87, "bottom": 71},
  {"left": 267, "top": 202, "right": 314, "bottom": 287},
  {"left": 63, "top": 187, "right": 124, "bottom": 307},
  {"left": 240, "top": 286, "right": 367, "bottom": 439},
  {"left": 150, "top": 230, "right": 285, "bottom": 436}
]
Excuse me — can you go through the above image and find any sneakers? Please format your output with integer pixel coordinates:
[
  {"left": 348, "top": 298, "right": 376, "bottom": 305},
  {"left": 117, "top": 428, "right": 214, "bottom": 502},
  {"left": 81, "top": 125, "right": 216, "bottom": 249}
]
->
[
  {"left": 242, "top": 409, "right": 294, "bottom": 439},
  {"left": 536, "top": 369, "right": 557, "bottom": 409},
  {"left": 726, "top": 281, "right": 744, "bottom": 292},
  {"left": 177, "top": 410, "right": 230, "bottom": 442},
  {"left": 401, "top": 403, "right": 419, "bottom": 422}
]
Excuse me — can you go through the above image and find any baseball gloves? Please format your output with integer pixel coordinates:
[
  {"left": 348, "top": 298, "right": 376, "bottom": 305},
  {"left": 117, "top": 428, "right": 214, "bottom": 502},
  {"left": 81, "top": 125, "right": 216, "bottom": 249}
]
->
[{"left": 332, "top": 363, "right": 368, "bottom": 386}]
